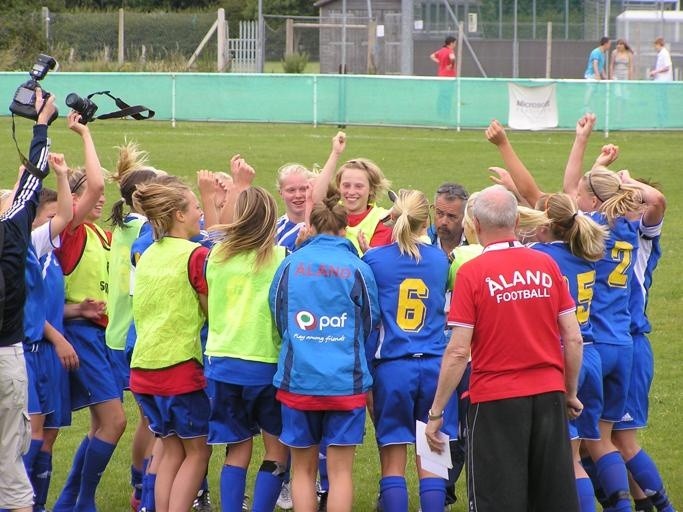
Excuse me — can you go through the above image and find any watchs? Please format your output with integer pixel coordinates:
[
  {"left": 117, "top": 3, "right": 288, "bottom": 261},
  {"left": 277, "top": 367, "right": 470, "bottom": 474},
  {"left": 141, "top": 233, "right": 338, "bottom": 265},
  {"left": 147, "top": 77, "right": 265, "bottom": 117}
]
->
[{"left": 428, "top": 409, "right": 444, "bottom": 420}]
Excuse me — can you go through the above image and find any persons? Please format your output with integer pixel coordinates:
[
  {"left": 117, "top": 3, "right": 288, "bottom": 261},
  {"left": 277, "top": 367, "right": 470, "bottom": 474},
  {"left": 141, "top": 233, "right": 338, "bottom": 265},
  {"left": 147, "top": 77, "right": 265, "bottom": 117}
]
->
[
  {"left": 103, "top": 137, "right": 158, "bottom": 511},
  {"left": 268, "top": 187, "right": 382, "bottom": 511},
  {"left": 57, "top": 165, "right": 126, "bottom": 510},
  {"left": 649, "top": 37, "right": 672, "bottom": 81},
  {"left": 431, "top": 36, "right": 457, "bottom": 77},
  {"left": 612, "top": 40, "right": 634, "bottom": 79},
  {"left": 133, "top": 175, "right": 210, "bottom": 510},
  {"left": 214, "top": 157, "right": 254, "bottom": 227},
  {"left": 426, "top": 186, "right": 582, "bottom": 510},
  {"left": 1, "top": 152, "right": 74, "bottom": 511},
  {"left": 0, "top": 86, "right": 57, "bottom": 511},
  {"left": 591, "top": 140, "right": 674, "bottom": 510},
  {"left": 205, "top": 186, "right": 292, "bottom": 511},
  {"left": 311, "top": 131, "right": 394, "bottom": 509},
  {"left": 360, "top": 190, "right": 460, "bottom": 510},
  {"left": 584, "top": 38, "right": 611, "bottom": 81},
  {"left": 274, "top": 164, "right": 324, "bottom": 510},
  {"left": 29, "top": 109, "right": 106, "bottom": 510},
  {"left": 487, "top": 118, "right": 641, "bottom": 509},
  {"left": 427, "top": 182, "right": 472, "bottom": 264},
  {"left": 489, "top": 168, "right": 602, "bottom": 511}
]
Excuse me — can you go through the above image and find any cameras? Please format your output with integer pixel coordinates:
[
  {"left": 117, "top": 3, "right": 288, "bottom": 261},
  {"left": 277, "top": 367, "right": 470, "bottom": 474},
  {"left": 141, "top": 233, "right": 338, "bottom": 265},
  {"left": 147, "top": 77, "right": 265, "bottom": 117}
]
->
[
  {"left": 9, "top": 52, "right": 60, "bottom": 179},
  {"left": 65, "top": 90, "right": 157, "bottom": 126}
]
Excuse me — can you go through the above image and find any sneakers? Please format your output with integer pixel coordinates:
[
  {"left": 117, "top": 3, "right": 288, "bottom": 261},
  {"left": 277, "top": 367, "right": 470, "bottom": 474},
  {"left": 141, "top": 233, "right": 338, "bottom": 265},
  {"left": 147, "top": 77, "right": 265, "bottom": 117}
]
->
[
  {"left": 241, "top": 493, "right": 250, "bottom": 510},
  {"left": 278, "top": 489, "right": 293, "bottom": 510},
  {"left": 130, "top": 488, "right": 140, "bottom": 512},
  {"left": 419, "top": 503, "right": 453, "bottom": 512},
  {"left": 194, "top": 489, "right": 215, "bottom": 511},
  {"left": 316, "top": 480, "right": 323, "bottom": 503}
]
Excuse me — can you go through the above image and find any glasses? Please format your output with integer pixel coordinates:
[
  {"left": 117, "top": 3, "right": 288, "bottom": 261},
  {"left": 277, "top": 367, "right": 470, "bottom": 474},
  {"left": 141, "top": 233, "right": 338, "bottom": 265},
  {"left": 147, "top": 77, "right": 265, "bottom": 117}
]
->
[{"left": 437, "top": 185, "right": 467, "bottom": 200}]
